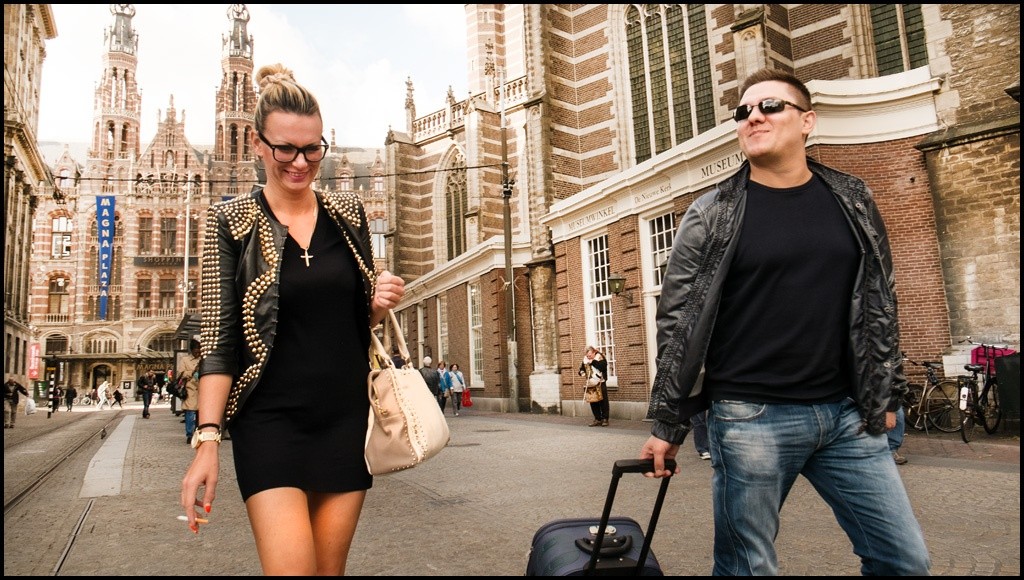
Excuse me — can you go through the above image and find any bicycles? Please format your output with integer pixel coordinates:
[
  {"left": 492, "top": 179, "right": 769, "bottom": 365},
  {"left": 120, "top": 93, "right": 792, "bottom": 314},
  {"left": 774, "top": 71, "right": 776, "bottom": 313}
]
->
[{"left": 895, "top": 336, "right": 1006, "bottom": 444}]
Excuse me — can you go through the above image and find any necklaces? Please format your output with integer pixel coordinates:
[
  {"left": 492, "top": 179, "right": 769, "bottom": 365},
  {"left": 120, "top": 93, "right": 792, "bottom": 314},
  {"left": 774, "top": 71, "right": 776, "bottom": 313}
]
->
[{"left": 264, "top": 194, "right": 315, "bottom": 267}]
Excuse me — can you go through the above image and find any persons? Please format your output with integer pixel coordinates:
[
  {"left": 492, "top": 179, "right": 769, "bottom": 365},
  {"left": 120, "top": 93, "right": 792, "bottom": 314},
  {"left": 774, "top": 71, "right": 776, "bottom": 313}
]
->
[
  {"left": 693, "top": 426, "right": 712, "bottom": 461},
  {"left": 578, "top": 346, "right": 609, "bottom": 427},
  {"left": 46, "top": 369, "right": 186, "bottom": 423},
  {"left": 390, "top": 346, "right": 404, "bottom": 367},
  {"left": 420, "top": 356, "right": 466, "bottom": 415},
  {"left": 182, "top": 62, "right": 405, "bottom": 576},
  {"left": 885, "top": 407, "right": 907, "bottom": 464},
  {"left": 178, "top": 339, "right": 201, "bottom": 444},
  {"left": 642, "top": 68, "right": 932, "bottom": 576},
  {"left": 4, "top": 377, "right": 29, "bottom": 428}
]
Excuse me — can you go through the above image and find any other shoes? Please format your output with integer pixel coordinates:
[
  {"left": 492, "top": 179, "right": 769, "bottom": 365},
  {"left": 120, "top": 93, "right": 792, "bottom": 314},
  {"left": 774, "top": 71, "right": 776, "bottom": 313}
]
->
[
  {"left": 588, "top": 419, "right": 602, "bottom": 426},
  {"left": 5, "top": 424, "right": 8, "bottom": 428},
  {"left": 10, "top": 425, "right": 14, "bottom": 428},
  {"left": 186, "top": 437, "right": 193, "bottom": 444},
  {"left": 602, "top": 419, "right": 609, "bottom": 426},
  {"left": 699, "top": 451, "right": 711, "bottom": 460}
]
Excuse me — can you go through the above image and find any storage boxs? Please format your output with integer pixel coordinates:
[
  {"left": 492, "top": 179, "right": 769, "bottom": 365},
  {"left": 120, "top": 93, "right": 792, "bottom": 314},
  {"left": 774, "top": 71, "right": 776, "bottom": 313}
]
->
[{"left": 972, "top": 346, "right": 1017, "bottom": 376}]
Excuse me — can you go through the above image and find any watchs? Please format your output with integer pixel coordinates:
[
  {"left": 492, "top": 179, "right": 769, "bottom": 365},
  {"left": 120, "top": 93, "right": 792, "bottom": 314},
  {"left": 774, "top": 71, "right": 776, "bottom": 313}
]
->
[{"left": 191, "top": 430, "right": 221, "bottom": 449}]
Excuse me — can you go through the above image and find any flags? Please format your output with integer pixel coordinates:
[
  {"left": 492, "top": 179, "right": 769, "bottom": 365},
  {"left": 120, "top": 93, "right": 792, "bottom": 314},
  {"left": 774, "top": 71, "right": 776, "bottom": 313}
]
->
[{"left": 96, "top": 196, "right": 115, "bottom": 319}]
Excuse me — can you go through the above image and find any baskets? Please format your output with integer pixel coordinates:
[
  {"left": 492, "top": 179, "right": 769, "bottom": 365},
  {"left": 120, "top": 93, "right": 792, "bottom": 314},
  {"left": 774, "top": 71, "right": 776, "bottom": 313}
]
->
[{"left": 972, "top": 347, "right": 1016, "bottom": 375}]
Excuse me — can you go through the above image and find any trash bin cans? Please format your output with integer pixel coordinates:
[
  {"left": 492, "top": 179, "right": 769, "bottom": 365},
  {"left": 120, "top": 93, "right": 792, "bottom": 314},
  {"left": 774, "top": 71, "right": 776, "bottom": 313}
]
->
[{"left": 996, "top": 352, "right": 1021, "bottom": 435}]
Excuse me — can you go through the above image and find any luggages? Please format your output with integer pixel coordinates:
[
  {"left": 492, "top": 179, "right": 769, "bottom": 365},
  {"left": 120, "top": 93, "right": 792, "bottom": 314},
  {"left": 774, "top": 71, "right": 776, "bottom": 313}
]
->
[{"left": 525, "top": 457, "right": 677, "bottom": 576}]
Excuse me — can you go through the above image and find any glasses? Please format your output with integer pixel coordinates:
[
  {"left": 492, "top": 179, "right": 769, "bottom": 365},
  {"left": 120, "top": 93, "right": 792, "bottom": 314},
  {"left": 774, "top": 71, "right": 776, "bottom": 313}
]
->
[
  {"left": 257, "top": 129, "right": 330, "bottom": 163},
  {"left": 732, "top": 98, "right": 807, "bottom": 123}
]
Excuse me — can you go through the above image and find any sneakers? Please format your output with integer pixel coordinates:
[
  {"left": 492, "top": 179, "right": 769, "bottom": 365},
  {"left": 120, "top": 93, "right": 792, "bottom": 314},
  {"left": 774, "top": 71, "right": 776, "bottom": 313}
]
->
[{"left": 891, "top": 449, "right": 909, "bottom": 464}]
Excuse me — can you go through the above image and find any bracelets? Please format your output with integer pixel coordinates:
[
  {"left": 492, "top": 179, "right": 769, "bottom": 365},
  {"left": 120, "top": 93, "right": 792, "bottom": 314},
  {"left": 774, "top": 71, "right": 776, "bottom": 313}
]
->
[{"left": 194, "top": 423, "right": 220, "bottom": 432}]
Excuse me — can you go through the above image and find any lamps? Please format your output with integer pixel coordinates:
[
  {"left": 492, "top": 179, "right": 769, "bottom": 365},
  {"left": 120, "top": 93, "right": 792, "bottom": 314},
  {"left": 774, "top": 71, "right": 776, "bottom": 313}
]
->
[{"left": 607, "top": 271, "right": 633, "bottom": 305}]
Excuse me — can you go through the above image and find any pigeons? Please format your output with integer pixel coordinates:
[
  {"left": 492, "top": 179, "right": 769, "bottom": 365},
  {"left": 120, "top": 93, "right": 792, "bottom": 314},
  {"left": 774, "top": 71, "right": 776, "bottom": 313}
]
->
[{"left": 100, "top": 426, "right": 107, "bottom": 439}]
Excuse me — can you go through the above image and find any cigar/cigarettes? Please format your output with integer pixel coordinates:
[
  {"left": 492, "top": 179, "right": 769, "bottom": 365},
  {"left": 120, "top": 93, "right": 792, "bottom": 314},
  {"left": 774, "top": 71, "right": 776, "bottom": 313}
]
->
[{"left": 177, "top": 516, "right": 208, "bottom": 523}]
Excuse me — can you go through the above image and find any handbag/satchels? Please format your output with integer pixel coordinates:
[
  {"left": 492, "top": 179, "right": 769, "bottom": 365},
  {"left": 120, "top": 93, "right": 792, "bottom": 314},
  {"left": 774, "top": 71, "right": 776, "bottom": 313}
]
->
[
  {"left": 166, "top": 382, "right": 188, "bottom": 400},
  {"left": 25, "top": 395, "right": 37, "bottom": 415},
  {"left": 462, "top": 389, "right": 472, "bottom": 407},
  {"left": 364, "top": 308, "right": 451, "bottom": 476},
  {"left": 583, "top": 382, "right": 603, "bottom": 403},
  {"left": 444, "top": 388, "right": 450, "bottom": 398}
]
